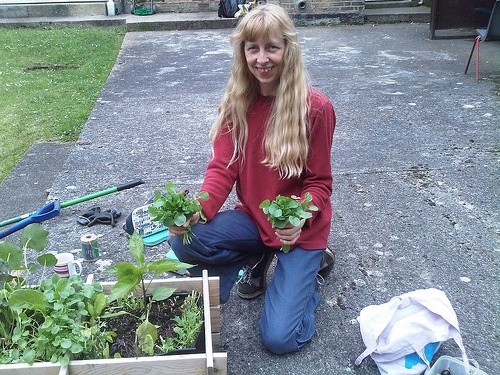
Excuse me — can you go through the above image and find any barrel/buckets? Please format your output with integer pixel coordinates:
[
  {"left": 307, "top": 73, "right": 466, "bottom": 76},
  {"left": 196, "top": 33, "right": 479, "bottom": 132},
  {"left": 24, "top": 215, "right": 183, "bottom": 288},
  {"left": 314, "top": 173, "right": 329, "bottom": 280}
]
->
[{"left": 80, "top": 234, "right": 99, "bottom": 261}]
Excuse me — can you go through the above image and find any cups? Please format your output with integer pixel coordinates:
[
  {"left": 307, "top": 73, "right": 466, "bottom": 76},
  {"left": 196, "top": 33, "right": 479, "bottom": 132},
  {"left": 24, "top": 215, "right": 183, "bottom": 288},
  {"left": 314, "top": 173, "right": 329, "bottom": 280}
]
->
[{"left": 53, "top": 253, "right": 82, "bottom": 279}]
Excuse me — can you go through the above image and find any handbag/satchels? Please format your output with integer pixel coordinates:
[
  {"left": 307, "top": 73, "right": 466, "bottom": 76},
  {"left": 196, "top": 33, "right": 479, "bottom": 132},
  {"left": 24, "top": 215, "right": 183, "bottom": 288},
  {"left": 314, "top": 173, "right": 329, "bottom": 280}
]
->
[{"left": 351, "top": 282, "right": 474, "bottom": 373}]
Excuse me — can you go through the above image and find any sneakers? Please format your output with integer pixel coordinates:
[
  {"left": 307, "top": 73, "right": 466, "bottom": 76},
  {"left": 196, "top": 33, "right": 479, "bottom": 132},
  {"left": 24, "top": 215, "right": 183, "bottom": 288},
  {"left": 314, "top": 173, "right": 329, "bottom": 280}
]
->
[
  {"left": 317, "top": 247, "right": 335, "bottom": 280},
  {"left": 235, "top": 249, "right": 275, "bottom": 298}
]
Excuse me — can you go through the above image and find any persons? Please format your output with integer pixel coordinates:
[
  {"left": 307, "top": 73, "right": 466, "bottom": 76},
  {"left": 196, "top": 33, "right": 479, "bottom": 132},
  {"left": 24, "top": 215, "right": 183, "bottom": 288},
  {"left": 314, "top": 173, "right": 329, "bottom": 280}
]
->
[{"left": 168, "top": 1, "right": 337, "bottom": 357}]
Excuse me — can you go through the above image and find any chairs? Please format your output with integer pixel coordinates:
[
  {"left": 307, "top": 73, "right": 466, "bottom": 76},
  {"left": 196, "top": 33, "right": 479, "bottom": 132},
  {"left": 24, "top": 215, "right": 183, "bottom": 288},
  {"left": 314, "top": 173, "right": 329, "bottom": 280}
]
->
[{"left": 464, "top": 0, "right": 500, "bottom": 82}]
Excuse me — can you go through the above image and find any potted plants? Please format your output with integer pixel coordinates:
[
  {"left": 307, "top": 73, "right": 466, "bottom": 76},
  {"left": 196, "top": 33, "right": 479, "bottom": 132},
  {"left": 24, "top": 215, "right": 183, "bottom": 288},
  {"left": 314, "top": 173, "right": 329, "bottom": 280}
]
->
[{"left": 0, "top": 222, "right": 228, "bottom": 375}]
